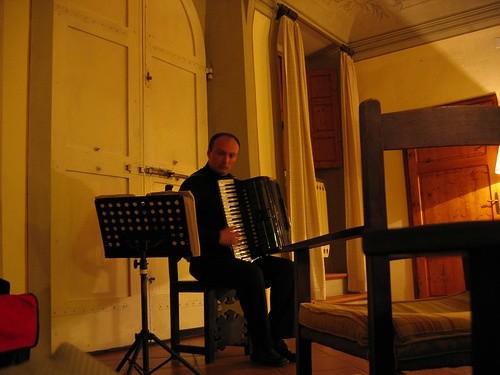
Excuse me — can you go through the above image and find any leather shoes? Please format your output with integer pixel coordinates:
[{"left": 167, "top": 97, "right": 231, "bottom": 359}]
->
[
  {"left": 271, "top": 341, "right": 297, "bottom": 362},
  {"left": 253, "top": 345, "right": 289, "bottom": 367}
]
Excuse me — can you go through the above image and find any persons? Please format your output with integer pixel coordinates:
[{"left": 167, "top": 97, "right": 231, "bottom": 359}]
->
[{"left": 179, "top": 133, "right": 298, "bottom": 366}]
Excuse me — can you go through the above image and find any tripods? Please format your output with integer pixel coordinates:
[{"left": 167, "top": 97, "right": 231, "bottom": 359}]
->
[{"left": 94, "top": 195, "right": 201, "bottom": 375}]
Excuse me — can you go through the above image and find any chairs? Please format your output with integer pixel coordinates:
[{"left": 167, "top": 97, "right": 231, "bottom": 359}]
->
[
  {"left": 165, "top": 184, "right": 253, "bottom": 364},
  {"left": 282, "top": 98, "right": 500, "bottom": 375}
]
